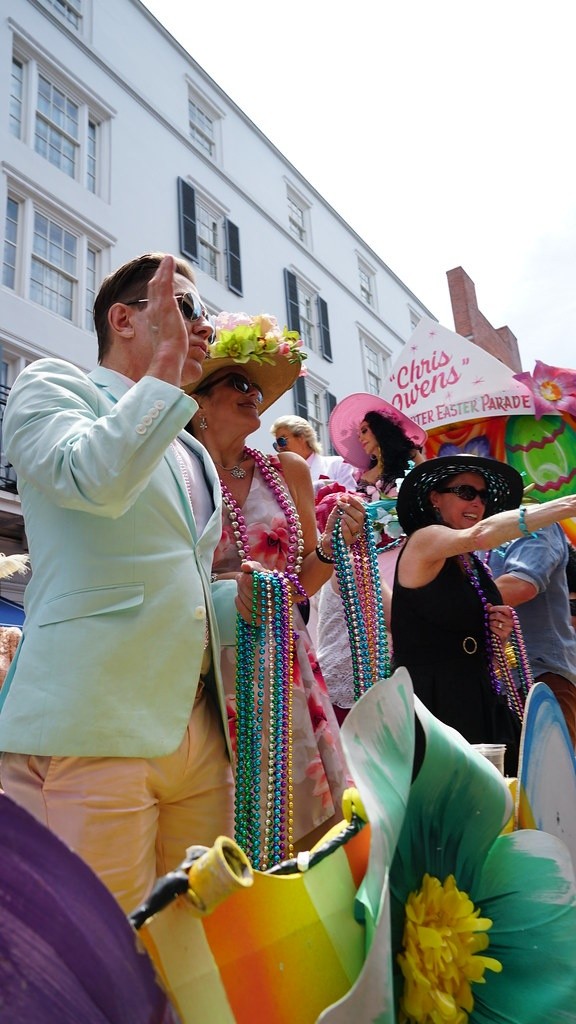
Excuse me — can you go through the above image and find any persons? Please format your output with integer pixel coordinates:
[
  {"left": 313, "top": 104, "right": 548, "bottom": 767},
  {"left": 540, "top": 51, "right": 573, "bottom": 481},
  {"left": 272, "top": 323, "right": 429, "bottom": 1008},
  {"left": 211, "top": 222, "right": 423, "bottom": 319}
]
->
[{"left": 0, "top": 256, "right": 574, "bottom": 915}]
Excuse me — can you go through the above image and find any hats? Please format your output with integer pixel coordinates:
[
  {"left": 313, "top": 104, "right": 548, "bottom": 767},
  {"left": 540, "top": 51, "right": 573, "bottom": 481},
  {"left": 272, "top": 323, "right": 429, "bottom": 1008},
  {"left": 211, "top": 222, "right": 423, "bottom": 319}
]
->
[
  {"left": 394, "top": 454, "right": 524, "bottom": 539},
  {"left": 327, "top": 392, "right": 428, "bottom": 471},
  {"left": 174, "top": 309, "right": 309, "bottom": 418}
]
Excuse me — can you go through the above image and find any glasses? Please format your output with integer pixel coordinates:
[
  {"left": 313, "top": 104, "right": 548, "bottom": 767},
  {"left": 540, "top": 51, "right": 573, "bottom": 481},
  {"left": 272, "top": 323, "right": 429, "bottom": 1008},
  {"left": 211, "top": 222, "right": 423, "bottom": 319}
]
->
[
  {"left": 191, "top": 372, "right": 264, "bottom": 404},
  {"left": 123, "top": 293, "right": 216, "bottom": 344},
  {"left": 439, "top": 485, "right": 491, "bottom": 506},
  {"left": 272, "top": 434, "right": 303, "bottom": 452}
]
